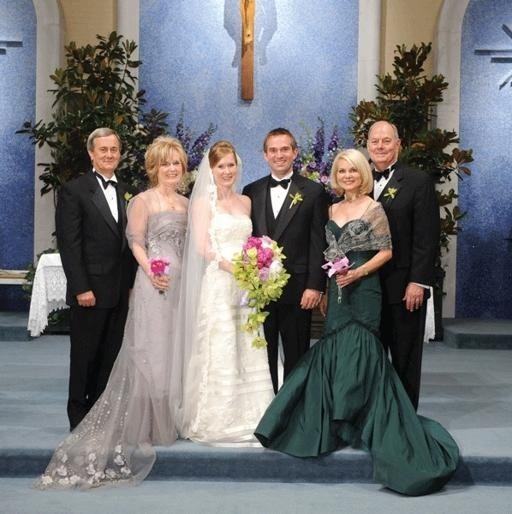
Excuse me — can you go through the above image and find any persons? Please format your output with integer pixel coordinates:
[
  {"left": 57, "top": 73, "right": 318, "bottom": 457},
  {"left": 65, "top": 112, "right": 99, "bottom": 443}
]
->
[
  {"left": 253, "top": 146, "right": 459, "bottom": 497},
  {"left": 169, "top": 139, "right": 283, "bottom": 447},
  {"left": 57, "top": 127, "right": 142, "bottom": 432},
  {"left": 368, "top": 122, "right": 440, "bottom": 412},
  {"left": 36, "top": 131, "right": 194, "bottom": 490},
  {"left": 242, "top": 128, "right": 330, "bottom": 398}
]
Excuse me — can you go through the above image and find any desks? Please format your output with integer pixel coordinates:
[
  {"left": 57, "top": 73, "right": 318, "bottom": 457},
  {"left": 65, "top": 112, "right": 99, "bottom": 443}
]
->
[{"left": 26, "top": 252, "right": 70, "bottom": 340}]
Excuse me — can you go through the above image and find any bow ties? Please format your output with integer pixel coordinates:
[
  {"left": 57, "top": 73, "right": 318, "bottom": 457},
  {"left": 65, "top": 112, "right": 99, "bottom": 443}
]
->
[
  {"left": 270, "top": 179, "right": 288, "bottom": 189},
  {"left": 374, "top": 169, "right": 389, "bottom": 182},
  {"left": 103, "top": 180, "right": 118, "bottom": 190}
]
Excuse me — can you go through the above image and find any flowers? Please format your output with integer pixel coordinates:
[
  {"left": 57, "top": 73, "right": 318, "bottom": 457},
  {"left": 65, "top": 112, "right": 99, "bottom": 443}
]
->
[
  {"left": 293, "top": 116, "right": 343, "bottom": 199},
  {"left": 232, "top": 236, "right": 290, "bottom": 350},
  {"left": 149, "top": 257, "right": 170, "bottom": 296},
  {"left": 322, "top": 257, "right": 355, "bottom": 304},
  {"left": 124, "top": 106, "right": 218, "bottom": 209}
]
